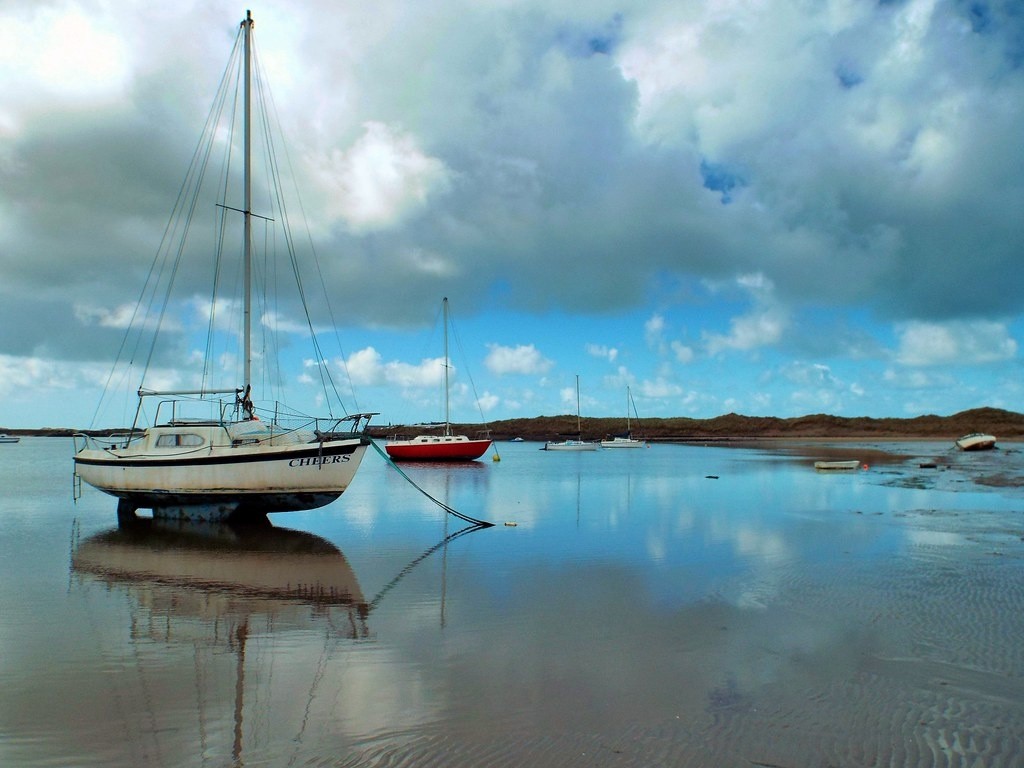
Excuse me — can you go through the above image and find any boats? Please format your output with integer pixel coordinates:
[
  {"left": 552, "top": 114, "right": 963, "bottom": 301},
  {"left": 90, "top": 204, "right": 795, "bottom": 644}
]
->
[
  {"left": 510, "top": 437, "right": 524, "bottom": 442},
  {"left": 539, "top": 439, "right": 600, "bottom": 451},
  {"left": 601, "top": 437, "right": 644, "bottom": 447},
  {"left": 0, "top": 434, "right": 20, "bottom": 443},
  {"left": 955, "top": 433, "right": 997, "bottom": 450},
  {"left": 815, "top": 461, "right": 861, "bottom": 468}
]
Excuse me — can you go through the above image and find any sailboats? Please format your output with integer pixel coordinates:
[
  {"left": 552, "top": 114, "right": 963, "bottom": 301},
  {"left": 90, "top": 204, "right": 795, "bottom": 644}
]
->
[
  {"left": 73, "top": 9, "right": 381, "bottom": 521},
  {"left": 70, "top": 520, "right": 370, "bottom": 768},
  {"left": 385, "top": 297, "right": 494, "bottom": 461}
]
